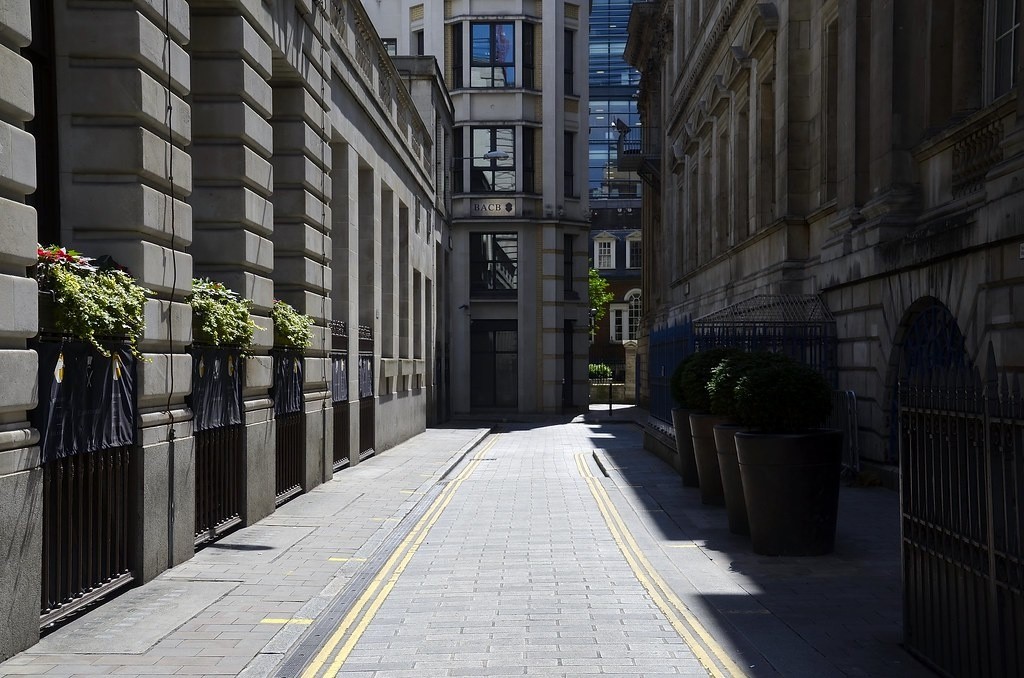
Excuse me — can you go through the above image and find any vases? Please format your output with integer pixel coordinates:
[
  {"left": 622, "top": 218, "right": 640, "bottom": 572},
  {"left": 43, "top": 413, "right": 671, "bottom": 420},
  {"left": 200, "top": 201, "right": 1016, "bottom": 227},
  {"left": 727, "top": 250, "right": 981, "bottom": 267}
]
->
[
  {"left": 273, "top": 324, "right": 306, "bottom": 349},
  {"left": 38, "top": 289, "right": 138, "bottom": 338},
  {"left": 192, "top": 312, "right": 241, "bottom": 346}
]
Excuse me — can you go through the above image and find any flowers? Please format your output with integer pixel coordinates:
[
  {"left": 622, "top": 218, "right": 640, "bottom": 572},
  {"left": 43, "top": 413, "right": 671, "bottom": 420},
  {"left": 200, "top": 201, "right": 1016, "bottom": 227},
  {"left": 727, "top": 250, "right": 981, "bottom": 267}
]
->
[
  {"left": 184, "top": 276, "right": 267, "bottom": 359},
  {"left": 26, "top": 242, "right": 159, "bottom": 364},
  {"left": 266, "top": 297, "right": 317, "bottom": 354}
]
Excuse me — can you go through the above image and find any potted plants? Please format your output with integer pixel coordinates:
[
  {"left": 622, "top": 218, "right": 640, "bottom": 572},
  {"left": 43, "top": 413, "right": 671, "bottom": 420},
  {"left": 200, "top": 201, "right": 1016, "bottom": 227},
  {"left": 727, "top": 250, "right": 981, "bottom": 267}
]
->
[
  {"left": 679, "top": 347, "right": 747, "bottom": 508},
  {"left": 668, "top": 353, "right": 696, "bottom": 483},
  {"left": 734, "top": 355, "right": 846, "bottom": 556},
  {"left": 704, "top": 351, "right": 792, "bottom": 537}
]
